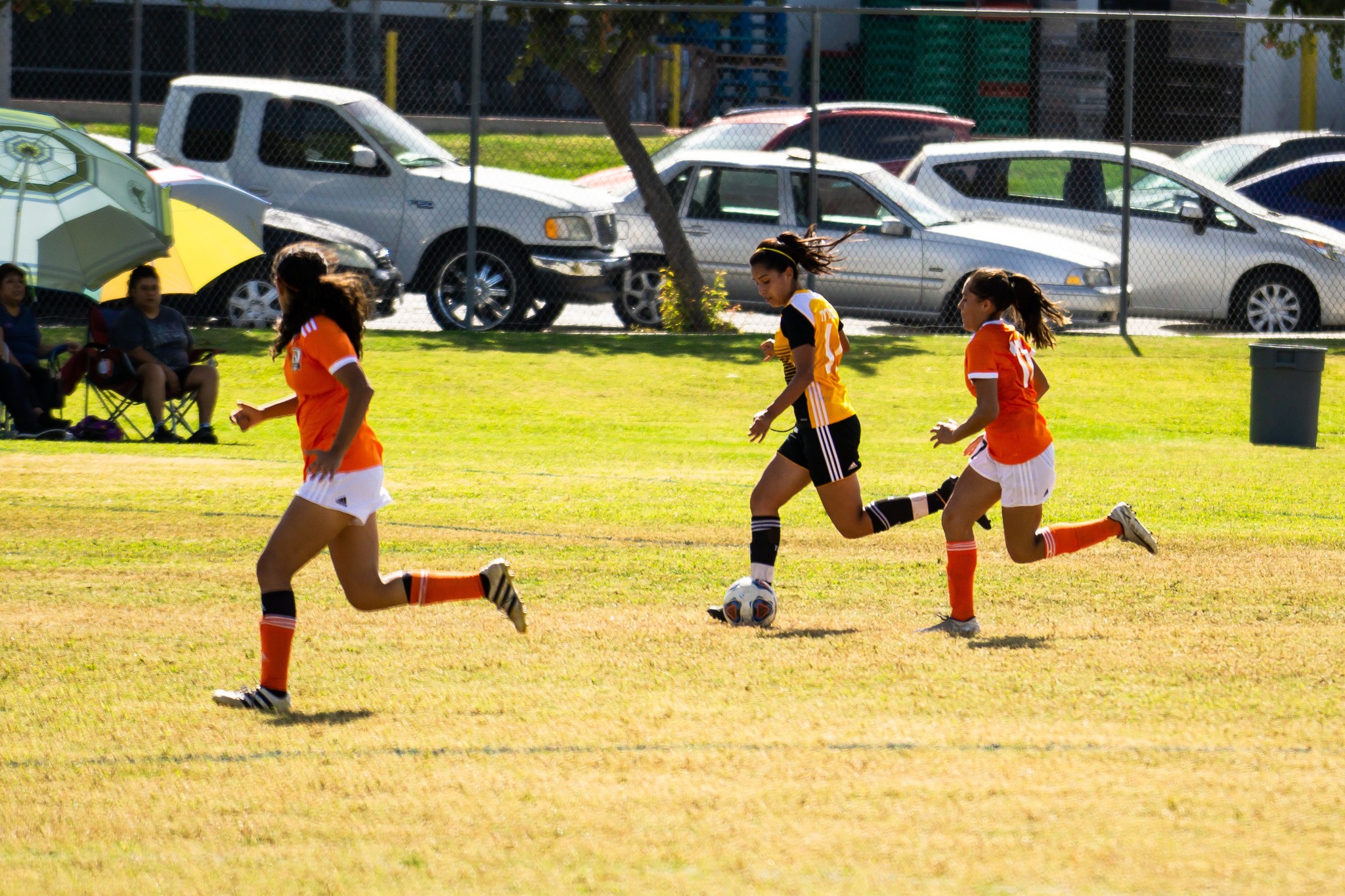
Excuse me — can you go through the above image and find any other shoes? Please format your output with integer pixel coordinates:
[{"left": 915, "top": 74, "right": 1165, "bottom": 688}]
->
[
  {"left": 187, "top": 426, "right": 218, "bottom": 445},
  {"left": 14, "top": 418, "right": 65, "bottom": 441},
  {"left": 37, "top": 412, "right": 71, "bottom": 431},
  {"left": 152, "top": 426, "right": 186, "bottom": 443}
]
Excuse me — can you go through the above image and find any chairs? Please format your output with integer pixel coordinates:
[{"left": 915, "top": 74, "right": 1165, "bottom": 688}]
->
[
  {"left": 668, "top": 181, "right": 706, "bottom": 219},
  {"left": 1064, "top": 173, "right": 1115, "bottom": 213},
  {"left": 85, "top": 304, "right": 227, "bottom": 441},
  {"left": 793, "top": 183, "right": 825, "bottom": 229},
  {"left": 939, "top": 161, "right": 1008, "bottom": 202}
]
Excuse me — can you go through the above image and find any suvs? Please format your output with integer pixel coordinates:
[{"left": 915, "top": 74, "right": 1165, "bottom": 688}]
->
[
  {"left": 156, "top": 76, "right": 632, "bottom": 336},
  {"left": 575, "top": 98, "right": 995, "bottom": 216}
]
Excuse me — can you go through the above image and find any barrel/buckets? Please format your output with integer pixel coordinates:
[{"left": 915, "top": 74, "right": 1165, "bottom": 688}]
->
[{"left": 1249, "top": 343, "right": 1329, "bottom": 448}]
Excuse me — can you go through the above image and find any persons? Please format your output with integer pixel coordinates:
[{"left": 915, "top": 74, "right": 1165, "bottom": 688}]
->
[
  {"left": 707, "top": 221, "right": 991, "bottom": 625},
  {"left": 919, "top": 268, "right": 1159, "bottom": 643},
  {"left": 212, "top": 241, "right": 529, "bottom": 715},
  {"left": 1, "top": 264, "right": 83, "bottom": 438},
  {"left": 106, "top": 265, "right": 217, "bottom": 444}
]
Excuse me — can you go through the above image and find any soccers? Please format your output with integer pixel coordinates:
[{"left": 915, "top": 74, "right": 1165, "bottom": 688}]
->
[{"left": 722, "top": 576, "right": 778, "bottom": 629}]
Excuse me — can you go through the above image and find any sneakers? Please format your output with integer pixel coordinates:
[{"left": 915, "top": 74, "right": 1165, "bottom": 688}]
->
[
  {"left": 707, "top": 607, "right": 727, "bottom": 623},
  {"left": 211, "top": 685, "right": 290, "bottom": 715},
  {"left": 479, "top": 557, "right": 529, "bottom": 634},
  {"left": 1106, "top": 502, "right": 1159, "bottom": 556},
  {"left": 937, "top": 475, "right": 991, "bottom": 530},
  {"left": 915, "top": 612, "right": 981, "bottom": 636}
]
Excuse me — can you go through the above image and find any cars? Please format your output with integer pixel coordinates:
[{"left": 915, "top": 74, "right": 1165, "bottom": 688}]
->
[
  {"left": 1105, "top": 130, "right": 1344, "bottom": 234},
  {"left": 871, "top": 137, "right": 1345, "bottom": 326},
  {"left": 590, "top": 149, "right": 1133, "bottom": 337},
  {"left": 88, "top": 133, "right": 406, "bottom": 339}
]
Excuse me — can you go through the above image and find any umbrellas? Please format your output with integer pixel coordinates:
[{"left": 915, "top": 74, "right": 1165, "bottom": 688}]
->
[
  {"left": 0, "top": 106, "right": 175, "bottom": 296},
  {"left": 82, "top": 165, "right": 272, "bottom": 304}
]
